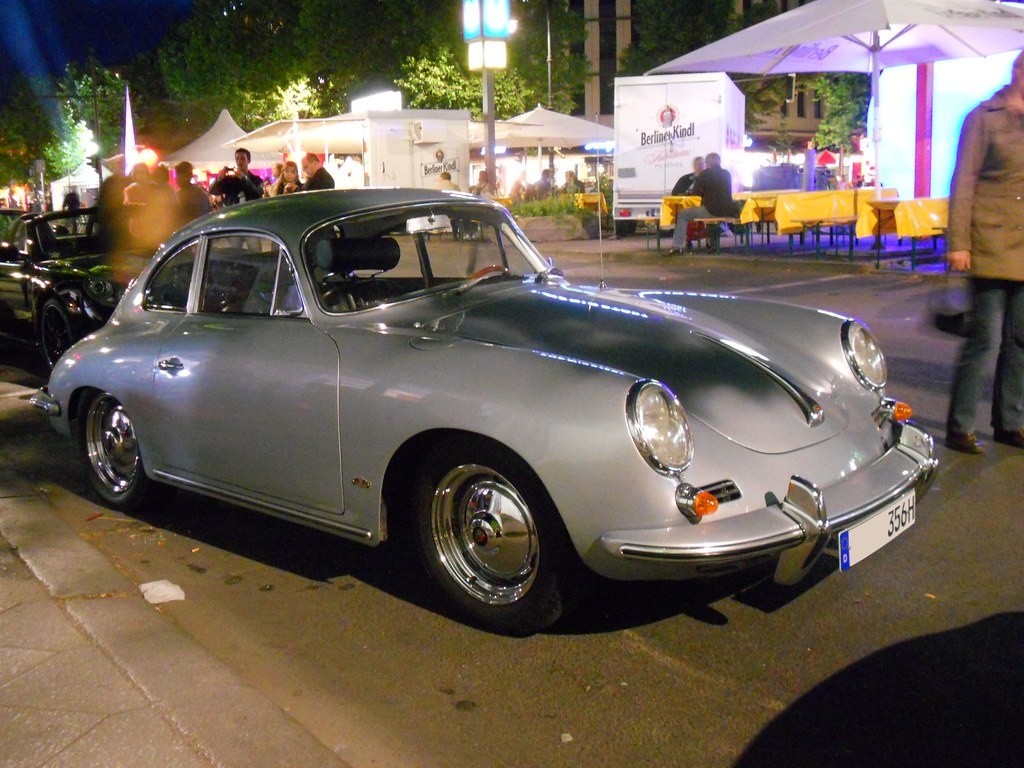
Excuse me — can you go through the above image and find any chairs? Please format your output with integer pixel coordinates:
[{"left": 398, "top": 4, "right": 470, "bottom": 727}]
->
[{"left": 342, "top": 237, "right": 407, "bottom": 310}]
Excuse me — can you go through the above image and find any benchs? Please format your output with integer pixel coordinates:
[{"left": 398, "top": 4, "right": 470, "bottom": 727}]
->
[
  {"left": 791, "top": 215, "right": 868, "bottom": 260},
  {"left": 932, "top": 226, "right": 952, "bottom": 273},
  {"left": 724, "top": 218, "right": 781, "bottom": 256},
  {"left": 830, "top": 218, "right": 869, "bottom": 259},
  {"left": 695, "top": 217, "right": 738, "bottom": 254},
  {"left": 646, "top": 217, "right": 666, "bottom": 251},
  {"left": 602, "top": 208, "right": 614, "bottom": 234}
]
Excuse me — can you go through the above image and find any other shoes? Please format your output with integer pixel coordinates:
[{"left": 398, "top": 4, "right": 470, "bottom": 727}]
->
[
  {"left": 663, "top": 248, "right": 682, "bottom": 257},
  {"left": 945, "top": 429, "right": 985, "bottom": 454},
  {"left": 706, "top": 246, "right": 716, "bottom": 254},
  {"left": 994, "top": 427, "right": 1024, "bottom": 448},
  {"left": 686, "top": 242, "right": 693, "bottom": 254}
]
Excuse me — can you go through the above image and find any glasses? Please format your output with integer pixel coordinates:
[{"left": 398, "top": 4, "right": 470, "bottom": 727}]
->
[{"left": 301, "top": 162, "right": 313, "bottom": 172}]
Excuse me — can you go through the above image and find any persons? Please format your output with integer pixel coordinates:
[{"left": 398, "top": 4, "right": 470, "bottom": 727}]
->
[
  {"left": 946, "top": 49, "right": 1024, "bottom": 453},
  {"left": 471, "top": 171, "right": 497, "bottom": 199},
  {"left": 509, "top": 168, "right": 586, "bottom": 200},
  {"left": 439, "top": 173, "right": 459, "bottom": 192},
  {"left": 62, "top": 147, "right": 336, "bottom": 249},
  {"left": 660, "top": 153, "right": 738, "bottom": 257}
]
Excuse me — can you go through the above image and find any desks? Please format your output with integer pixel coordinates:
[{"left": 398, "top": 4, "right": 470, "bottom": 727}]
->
[
  {"left": 492, "top": 192, "right": 608, "bottom": 216},
  {"left": 660, "top": 188, "right": 802, "bottom": 255},
  {"left": 740, "top": 188, "right": 901, "bottom": 259},
  {"left": 866, "top": 196, "right": 950, "bottom": 273}
]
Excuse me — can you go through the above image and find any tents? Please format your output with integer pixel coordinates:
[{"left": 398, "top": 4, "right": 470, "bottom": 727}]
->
[
  {"left": 220, "top": 107, "right": 545, "bottom": 171},
  {"left": 168, "top": 109, "right": 272, "bottom": 162}
]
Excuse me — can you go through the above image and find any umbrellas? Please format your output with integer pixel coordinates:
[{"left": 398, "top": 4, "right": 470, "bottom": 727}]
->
[
  {"left": 470, "top": 102, "right": 615, "bottom": 179},
  {"left": 643, "top": 0, "right": 1024, "bottom": 202}
]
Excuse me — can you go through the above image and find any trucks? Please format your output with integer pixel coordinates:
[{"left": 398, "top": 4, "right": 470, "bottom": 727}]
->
[{"left": 607, "top": 71, "right": 746, "bottom": 250}]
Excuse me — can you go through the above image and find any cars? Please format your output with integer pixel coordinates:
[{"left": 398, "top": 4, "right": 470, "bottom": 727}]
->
[
  {"left": 36, "top": 188, "right": 936, "bottom": 640},
  {"left": 0, "top": 209, "right": 159, "bottom": 373}
]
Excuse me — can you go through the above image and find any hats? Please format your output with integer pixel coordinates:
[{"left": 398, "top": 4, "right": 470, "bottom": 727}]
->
[
  {"left": 152, "top": 164, "right": 170, "bottom": 182},
  {"left": 175, "top": 161, "right": 193, "bottom": 178}
]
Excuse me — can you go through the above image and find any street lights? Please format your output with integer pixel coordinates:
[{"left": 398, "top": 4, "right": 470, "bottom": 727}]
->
[{"left": 462, "top": 1, "right": 511, "bottom": 191}]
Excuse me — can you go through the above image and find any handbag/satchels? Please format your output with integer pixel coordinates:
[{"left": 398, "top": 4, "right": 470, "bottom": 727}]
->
[{"left": 934, "top": 267, "right": 975, "bottom": 339}]
